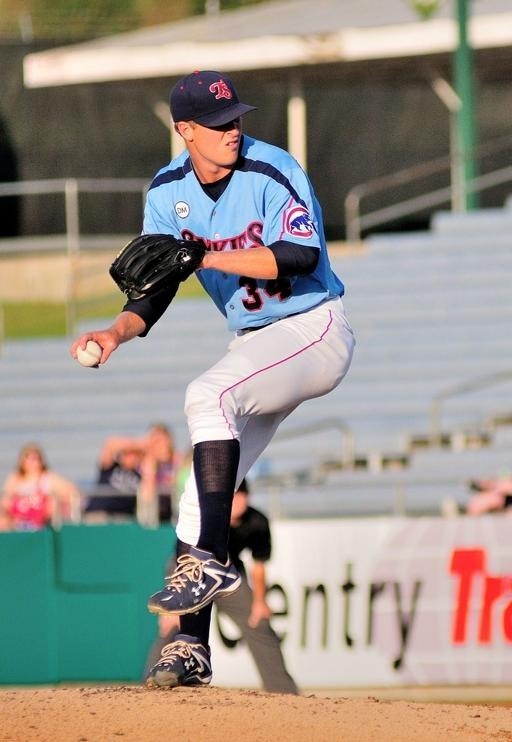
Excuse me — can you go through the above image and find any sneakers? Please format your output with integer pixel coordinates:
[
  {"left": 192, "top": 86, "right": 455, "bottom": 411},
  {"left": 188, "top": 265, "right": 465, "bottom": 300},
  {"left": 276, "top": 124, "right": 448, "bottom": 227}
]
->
[
  {"left": 145, "top": 546, "right": 242, "bottom": 615},
  {"left": 142, "top": 634, "right": 213, "bottom": 688}
]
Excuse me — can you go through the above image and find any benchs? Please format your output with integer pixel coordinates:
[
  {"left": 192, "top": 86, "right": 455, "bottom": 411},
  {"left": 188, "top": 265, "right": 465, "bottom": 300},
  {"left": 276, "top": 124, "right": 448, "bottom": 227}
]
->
[
  {"left": 163, "top": 209, "right": 512, "bottom": 317},
  {"left": 0, "top": 317, "right": 512, "bottom": 520}
]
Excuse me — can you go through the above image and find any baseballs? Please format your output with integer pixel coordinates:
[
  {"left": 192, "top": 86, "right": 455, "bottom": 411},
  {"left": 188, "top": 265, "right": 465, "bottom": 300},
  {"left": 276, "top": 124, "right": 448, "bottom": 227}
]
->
[{"left": 76, "top": 341, "right": 102, "bottom": 367}]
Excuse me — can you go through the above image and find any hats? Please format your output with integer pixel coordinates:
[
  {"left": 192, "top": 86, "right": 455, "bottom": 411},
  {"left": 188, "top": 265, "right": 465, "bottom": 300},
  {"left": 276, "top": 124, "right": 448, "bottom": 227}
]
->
[
  {"left": 238, "top": 476, "right": 249, "bottom": 493},
  {"left": 170, "top": 71, "right": 259, "bottom": 128}
]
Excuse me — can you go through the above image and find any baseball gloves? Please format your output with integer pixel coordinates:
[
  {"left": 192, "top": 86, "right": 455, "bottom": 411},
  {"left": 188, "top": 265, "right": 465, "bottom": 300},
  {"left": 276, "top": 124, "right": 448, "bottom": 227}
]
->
[{"left": 109, "top": 233, "right": 206, "bottom": 302}]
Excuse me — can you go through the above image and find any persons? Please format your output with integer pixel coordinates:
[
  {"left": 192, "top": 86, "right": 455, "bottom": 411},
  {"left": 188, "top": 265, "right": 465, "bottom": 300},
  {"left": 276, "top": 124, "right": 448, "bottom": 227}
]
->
[
  {"left": 143, "top": 474, "right": 301, "bottom": 697},
  {"left": 84, "top": 435, "right": 153, "bottom": 526},
  {"left": 0, "top": 443, "right": 86, "bottom": 528},
  {"left": 135, "top": 421, "right": 187, "bottom": 528},
  {"left": 460, "top": 476, "right": 511, "bottom": 515},
  {"left": 66, "top": 67, "right": 360, "bottom": 688},
  {"left": 169, "top": 446, "right": 194, "bottom": 521}
]
